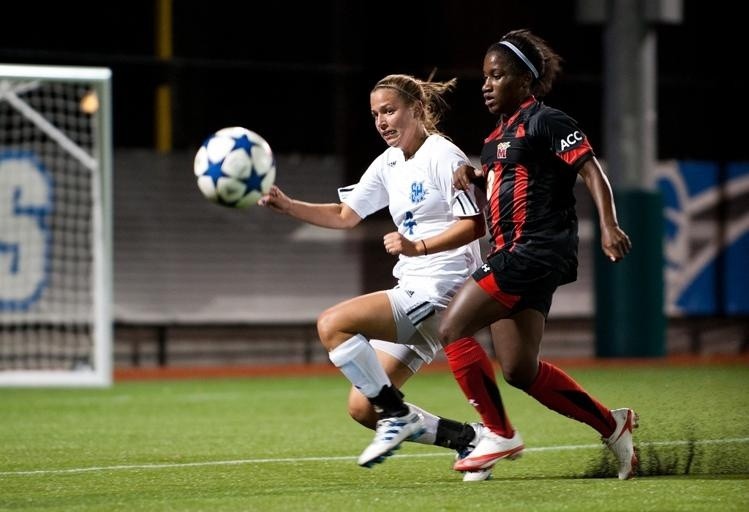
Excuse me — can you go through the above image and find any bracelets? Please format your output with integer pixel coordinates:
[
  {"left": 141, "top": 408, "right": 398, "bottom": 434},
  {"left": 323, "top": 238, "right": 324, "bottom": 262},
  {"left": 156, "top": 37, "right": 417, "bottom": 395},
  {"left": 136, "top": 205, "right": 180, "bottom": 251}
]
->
[{"left": 421, "top": 239, "right": 428, "bottom": 256}]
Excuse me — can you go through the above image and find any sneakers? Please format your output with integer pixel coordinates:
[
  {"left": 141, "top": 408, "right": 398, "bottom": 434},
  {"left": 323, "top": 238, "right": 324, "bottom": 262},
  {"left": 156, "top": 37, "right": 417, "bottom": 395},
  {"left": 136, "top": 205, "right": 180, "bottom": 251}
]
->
[
  {"left": 455, "top": 421, "right": 496, "bottom": 483},
  {"left": 600, "top": 407, "right": 641, "bottom": 482},
  {"left": 357, "top": 406, "right": 428, "bottom": 468},
  {"left": 453, "top": 428, "right": 527, "bottom": 472}
]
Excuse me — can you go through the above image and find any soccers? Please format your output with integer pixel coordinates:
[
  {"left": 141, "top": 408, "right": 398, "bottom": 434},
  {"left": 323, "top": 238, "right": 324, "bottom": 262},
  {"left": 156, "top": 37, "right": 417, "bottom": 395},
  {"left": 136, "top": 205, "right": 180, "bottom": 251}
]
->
[{"left": 194, "top": 126, "right": 275, "bottom": 210}]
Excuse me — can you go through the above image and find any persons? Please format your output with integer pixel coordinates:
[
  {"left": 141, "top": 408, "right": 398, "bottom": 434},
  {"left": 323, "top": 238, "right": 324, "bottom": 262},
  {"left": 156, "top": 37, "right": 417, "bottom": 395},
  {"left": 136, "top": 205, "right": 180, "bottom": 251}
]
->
[
  {"left": 257, "top": 74, "right": 494, "bottom": 481},
  {"left": 436, "top": 28, "right": 637, "bottom": 480}
]
